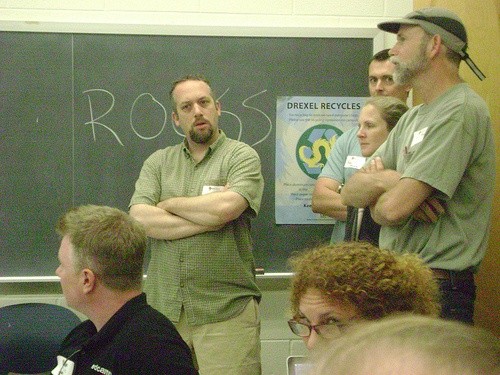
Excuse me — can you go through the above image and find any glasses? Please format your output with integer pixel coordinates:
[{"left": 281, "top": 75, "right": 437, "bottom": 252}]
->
[{"left": 287, "top": 312, "right": 365, "bottom": 340}]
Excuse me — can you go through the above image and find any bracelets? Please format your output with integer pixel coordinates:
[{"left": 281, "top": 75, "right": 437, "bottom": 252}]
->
[{"left": 337, "top": 183, "right": 345, "bottom": 193}]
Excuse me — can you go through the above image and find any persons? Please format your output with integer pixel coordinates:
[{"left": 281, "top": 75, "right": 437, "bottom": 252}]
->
[
  {"left": 287, "top": 241, "right": 440, "bottom": 363},
  {"left": 127, "top": 73, "right": 264, "bottom": 375},
  {"left": 41, "top": 202, "right": 202, "bottom": 375},
  {"left": 311, "top": 47, "right": 412, "bottom": 247},
  {"left": 340, "top": 6, "right": 495, "bottom": 328},
  {"left": 340, "top": 95, "right": 410, "bottom": 251}
]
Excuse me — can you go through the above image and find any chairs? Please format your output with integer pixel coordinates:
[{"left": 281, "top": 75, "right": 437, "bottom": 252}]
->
[{"left": 0, "top": 303, "right": 82, "bottom": 375}]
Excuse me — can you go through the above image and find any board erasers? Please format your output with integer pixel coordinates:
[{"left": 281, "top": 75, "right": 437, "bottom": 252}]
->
[{"left": 254, "top": 268, "right": 265, "bottom": 275}]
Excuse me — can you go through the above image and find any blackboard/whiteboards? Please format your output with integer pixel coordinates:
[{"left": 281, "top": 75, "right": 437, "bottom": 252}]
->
[{"left": 0, "top": 24, "right": 390, "bottom": 286}]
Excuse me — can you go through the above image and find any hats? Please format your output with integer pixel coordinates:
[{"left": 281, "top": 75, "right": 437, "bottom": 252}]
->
[{"left": 377, "top": 9, "right": 486, "bottom": 81}]
